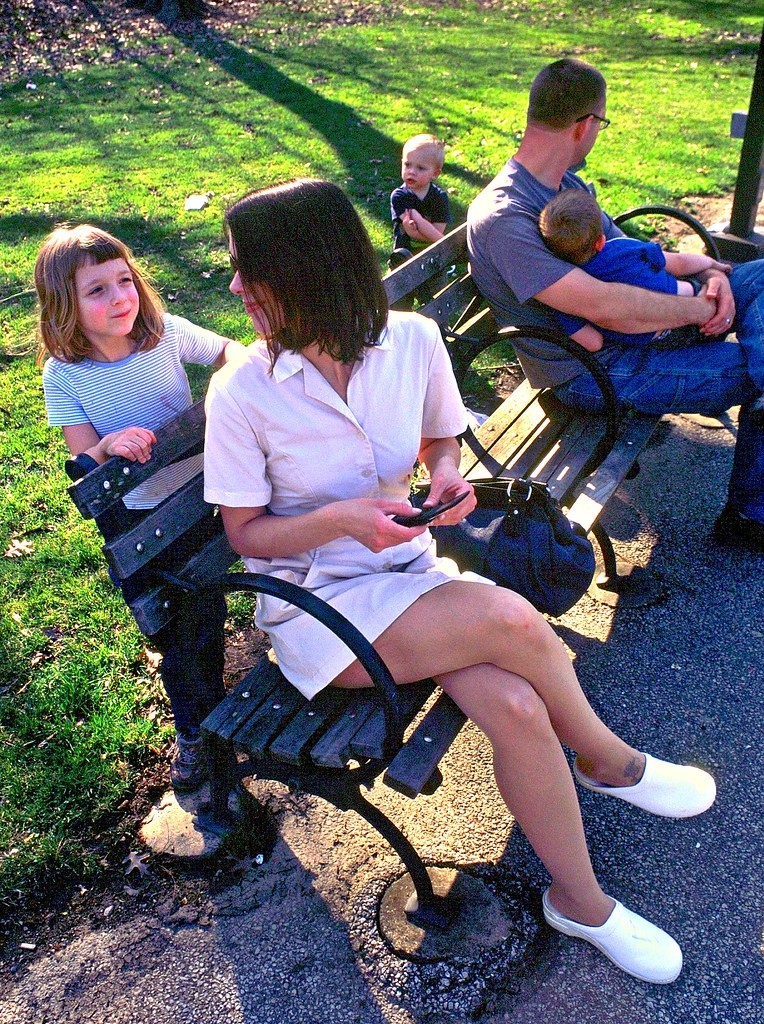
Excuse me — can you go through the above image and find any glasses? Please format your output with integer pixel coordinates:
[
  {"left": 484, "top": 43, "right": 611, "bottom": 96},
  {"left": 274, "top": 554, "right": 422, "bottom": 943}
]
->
[
  {"left": 577, "top": 113, "right": 610, "bottom": 130},
  {"left": 229, "top": 252, "right": 269, "bottom": 283}
]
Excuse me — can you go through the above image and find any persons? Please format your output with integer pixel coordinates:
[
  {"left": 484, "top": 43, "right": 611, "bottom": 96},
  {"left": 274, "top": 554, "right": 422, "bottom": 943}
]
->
[
  {"left": 387, "top": 133, "right": 451, "bottom": 309},
  {"left": 540, "top": 188, "right": 735, "bottom": 353},
  {"left": 467, "top": 56, "right": 764, "bottom": 554},
  {"left": 32, "top": 224, "right": 246, "bottom": 790},
  {"left": 202, "top": 181, "right": 716, "bottom": 986}
]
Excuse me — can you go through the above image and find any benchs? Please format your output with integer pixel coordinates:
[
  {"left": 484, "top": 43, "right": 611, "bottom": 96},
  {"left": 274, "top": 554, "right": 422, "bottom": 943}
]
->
[{"left": 65, "top": 207, "right": 719, "bottom": 957}]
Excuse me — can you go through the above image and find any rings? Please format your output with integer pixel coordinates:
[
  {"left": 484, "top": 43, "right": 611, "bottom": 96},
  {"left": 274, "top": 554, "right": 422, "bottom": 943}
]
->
[{"left": 726, "top": 318, "right": 730, "bottom": 323}]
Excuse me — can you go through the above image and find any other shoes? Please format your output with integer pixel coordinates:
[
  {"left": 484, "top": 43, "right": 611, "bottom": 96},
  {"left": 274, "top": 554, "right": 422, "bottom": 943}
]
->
[
  {"left": 717, "top": 504, "right": 763, "bottom": 551},
  {"left": 170, "top": 732, "right": 203, "bottom": 789}
]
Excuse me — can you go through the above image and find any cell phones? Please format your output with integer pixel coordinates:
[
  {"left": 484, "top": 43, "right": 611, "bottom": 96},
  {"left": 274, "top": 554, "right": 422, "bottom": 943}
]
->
[{"left": 392, "top": 490, "right": 470, "bottom": 527}]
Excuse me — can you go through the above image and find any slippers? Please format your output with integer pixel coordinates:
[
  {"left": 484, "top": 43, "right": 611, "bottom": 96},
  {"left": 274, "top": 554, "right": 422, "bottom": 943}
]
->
[
  {"left": 544, "top": 886, "right": 683, "bottom": 985},
  {"left": 573, "top": 753, "right": 717, "bottom": 817}
]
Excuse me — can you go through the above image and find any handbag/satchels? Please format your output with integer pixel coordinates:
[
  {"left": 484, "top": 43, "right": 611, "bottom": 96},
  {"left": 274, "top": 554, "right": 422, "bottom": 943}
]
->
[{"left": 411, "top": 477, "right": 595, "bottom": 618}]
